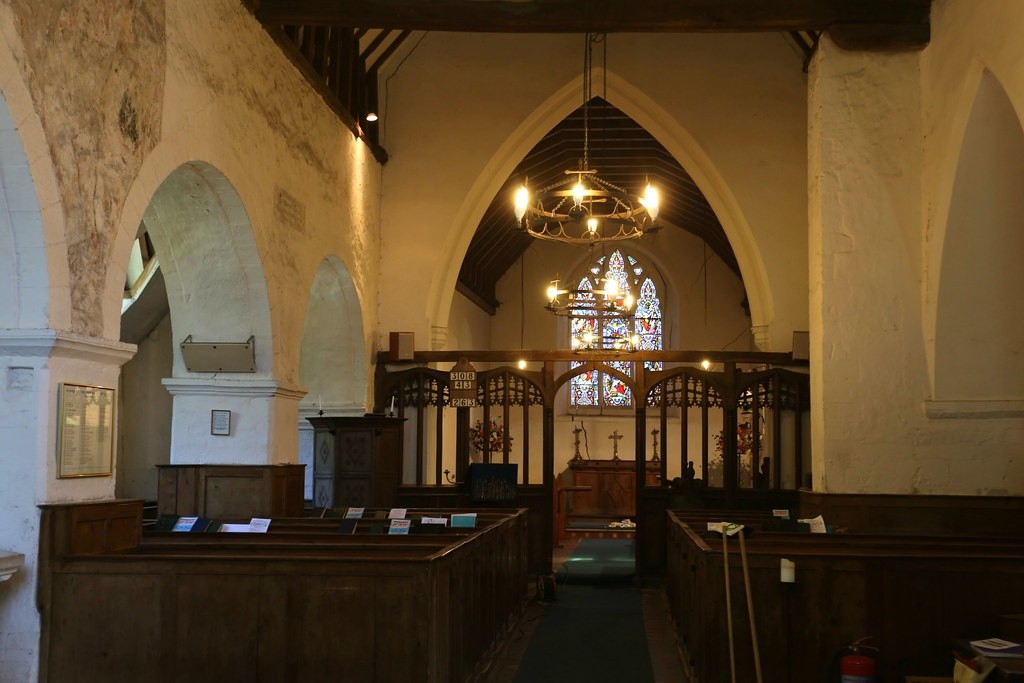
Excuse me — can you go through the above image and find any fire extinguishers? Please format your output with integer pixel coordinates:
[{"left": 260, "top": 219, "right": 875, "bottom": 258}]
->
[{"left": 826, "top": 636, "right": 880, "bottom": 683}]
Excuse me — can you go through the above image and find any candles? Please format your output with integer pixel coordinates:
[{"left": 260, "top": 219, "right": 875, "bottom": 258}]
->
[
  {"left": 319, "top": 395, "right": 321, "bottom": 410},
  {"left": 391, "top": 396, "right": 395, "bottom": 412}
]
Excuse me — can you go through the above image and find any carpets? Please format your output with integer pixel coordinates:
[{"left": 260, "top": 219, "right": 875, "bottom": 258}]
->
[{"left": 512, "top": 537, "right": 655, "bottom": 683}]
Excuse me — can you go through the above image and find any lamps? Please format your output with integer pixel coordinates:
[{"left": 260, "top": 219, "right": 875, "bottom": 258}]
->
[
  {"left": 543, "top": 221, "right": 636, "bottom": 319},
  {"left": 511, "top": 0, "right": 664, "bottom": 248},
  {"left": 366, "top": 67, "right": 379, "bottom": 120}
]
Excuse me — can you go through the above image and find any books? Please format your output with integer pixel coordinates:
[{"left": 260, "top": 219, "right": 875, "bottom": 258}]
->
[
  {"left": 707, "top": 522, "right": 752, "bottom": 540},
  {"left": 762, "top": 510, "right": 828, "bottom": 533},
  {"left": 155, "top": 514, "right": 272, "bottom": 533},
  {"left": 338, "top": 507, "right": 478, "bottom": 534},
  {"left": 970, "top": 638, "right": 1024, "bottom": 659}
]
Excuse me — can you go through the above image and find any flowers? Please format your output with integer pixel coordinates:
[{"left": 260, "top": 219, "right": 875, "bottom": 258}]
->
[
  {"left": 470, "top": 416, "right": 514, "bottom": 464},
  {"left": 711, "top": 422, "right": 764, "bottom": 487}
]
